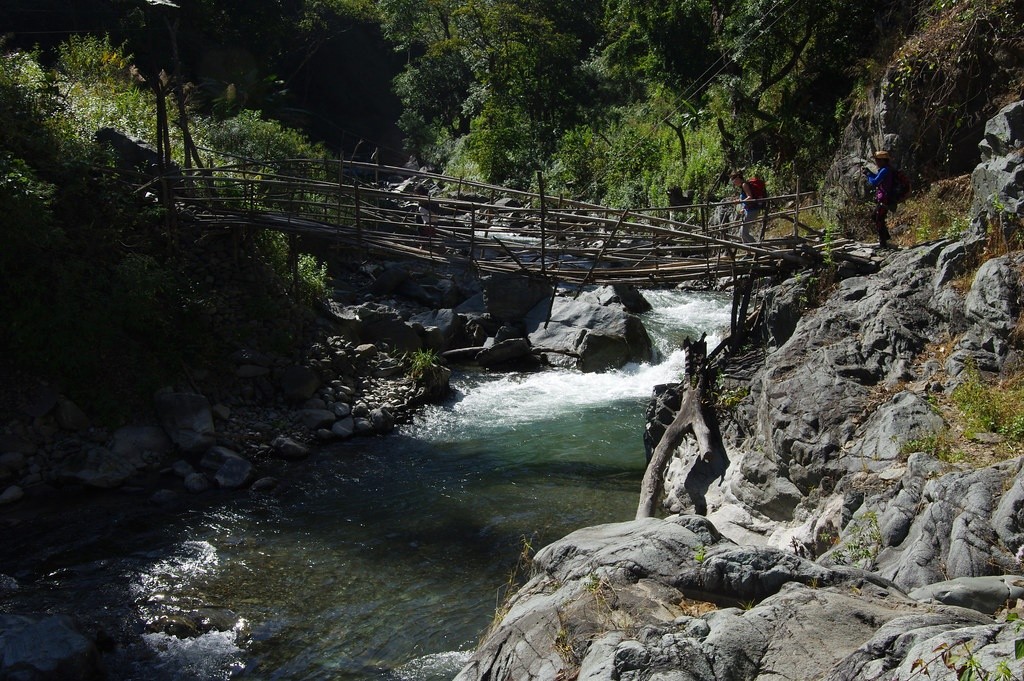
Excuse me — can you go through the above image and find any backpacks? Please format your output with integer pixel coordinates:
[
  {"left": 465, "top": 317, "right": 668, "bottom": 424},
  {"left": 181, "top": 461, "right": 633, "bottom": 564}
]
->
[
  {"left": 878, "top": 166, "right": 912, "bottom": 203},
  {"left": 740, "top": 177, "right": 767, "bottom": 209}
]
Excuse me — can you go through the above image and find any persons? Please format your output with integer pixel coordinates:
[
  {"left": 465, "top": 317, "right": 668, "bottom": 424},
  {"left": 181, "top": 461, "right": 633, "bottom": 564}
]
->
[
  {"left": 730, "top": 170, "right": 768, "bottom": 263},
  {"left": 861, "top": 150, "right": 899, "bottom": 249}
]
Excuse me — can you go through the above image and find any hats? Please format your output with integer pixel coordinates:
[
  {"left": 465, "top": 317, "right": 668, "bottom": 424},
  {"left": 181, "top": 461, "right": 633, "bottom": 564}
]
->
[{"left": 870, "top": 150, "right": 892, "bottom": 159}]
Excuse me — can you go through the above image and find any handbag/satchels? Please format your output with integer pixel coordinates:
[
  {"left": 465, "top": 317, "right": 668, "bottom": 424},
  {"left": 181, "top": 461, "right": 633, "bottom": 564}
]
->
[
  {"left": 877, "top": 184, "right": 887, "bottom": 204},
  {"left": 736, "top": 204, "right": 745, "bottom": 216}
]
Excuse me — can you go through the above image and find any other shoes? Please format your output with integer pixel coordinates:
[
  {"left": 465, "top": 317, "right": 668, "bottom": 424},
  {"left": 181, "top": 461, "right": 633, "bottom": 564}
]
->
[{"left": 873, "top": 244, "right": 889, "bottom": 250}]
